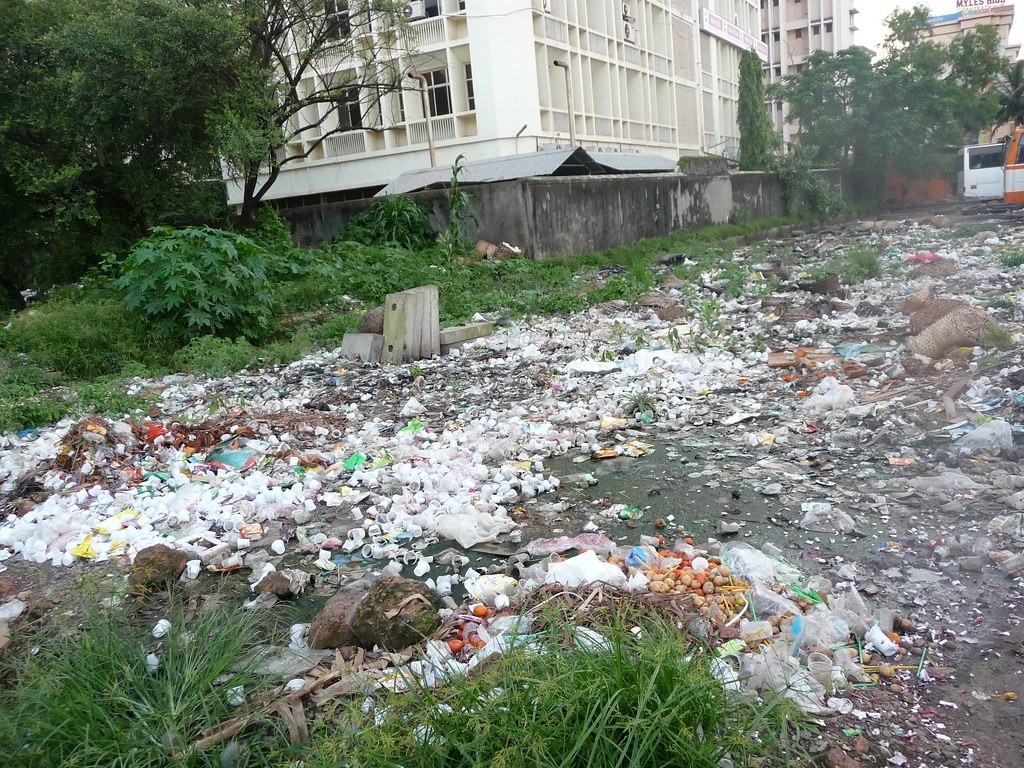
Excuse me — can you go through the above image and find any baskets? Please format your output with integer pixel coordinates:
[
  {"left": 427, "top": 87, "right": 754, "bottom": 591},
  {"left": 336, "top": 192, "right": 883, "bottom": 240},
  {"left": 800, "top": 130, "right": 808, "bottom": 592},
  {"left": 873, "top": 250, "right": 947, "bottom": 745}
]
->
[
  {"left": 910, "top": 308, "right": 996, "bottom": 358},
  {"left": 908, "top": 298, "right": 962, "bottom": 336}
]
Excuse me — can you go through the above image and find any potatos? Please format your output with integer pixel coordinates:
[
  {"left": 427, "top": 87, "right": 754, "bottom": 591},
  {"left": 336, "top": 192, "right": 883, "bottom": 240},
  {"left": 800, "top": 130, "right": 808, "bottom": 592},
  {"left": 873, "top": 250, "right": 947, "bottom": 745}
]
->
[
  {"left": 647, "top": 563, "right": 814, "bottom": 634},
  {"left": 845, "top": 619, "right": 956, "bottom": 692}
]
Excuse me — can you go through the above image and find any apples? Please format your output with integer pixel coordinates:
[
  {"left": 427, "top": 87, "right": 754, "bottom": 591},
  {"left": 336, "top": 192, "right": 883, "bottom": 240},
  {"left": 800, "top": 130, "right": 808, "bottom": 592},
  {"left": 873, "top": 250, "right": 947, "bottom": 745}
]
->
[
  {"left": 656, "top": 535, "right": 720, "bottom": 597},
  {"left": 447, "top": 604, "right": 487, "bottom": 653}
]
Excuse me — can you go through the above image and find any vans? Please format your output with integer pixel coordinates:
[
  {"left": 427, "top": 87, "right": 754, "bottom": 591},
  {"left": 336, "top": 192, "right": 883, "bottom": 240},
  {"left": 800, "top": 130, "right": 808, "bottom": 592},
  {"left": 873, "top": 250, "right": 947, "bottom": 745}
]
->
[
  {"left": 955, "top": 140, "right": 1024, "bottom": 203},
  {"left": 1000, "top": 125, "right": 1024, "bottom": 204}
]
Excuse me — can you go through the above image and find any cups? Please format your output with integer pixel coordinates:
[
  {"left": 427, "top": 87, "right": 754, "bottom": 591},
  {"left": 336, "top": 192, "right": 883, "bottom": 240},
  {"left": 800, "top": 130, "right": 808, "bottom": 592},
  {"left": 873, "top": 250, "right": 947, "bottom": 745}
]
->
[{"left": 0, "top": 413, "right": 1023, "bottom": 710}]
